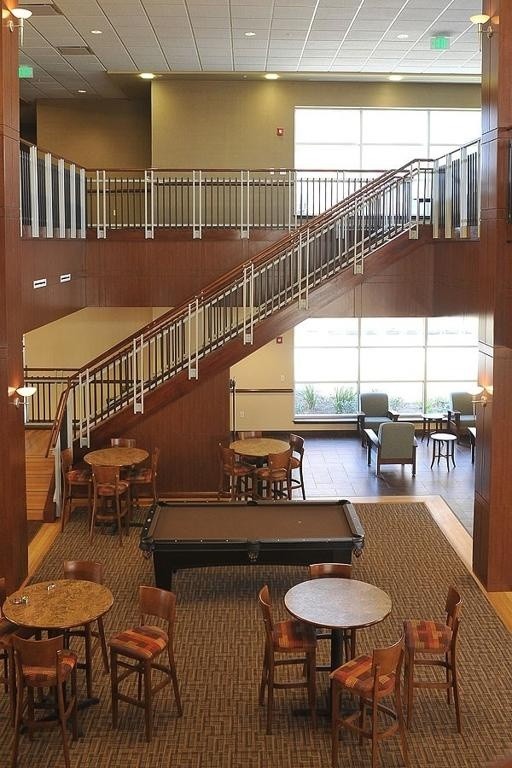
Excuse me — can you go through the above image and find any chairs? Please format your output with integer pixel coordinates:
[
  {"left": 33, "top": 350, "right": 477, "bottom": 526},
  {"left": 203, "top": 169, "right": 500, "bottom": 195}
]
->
[
  {"left": 355, "top": 390, "right": 402, "bottom": 440},
  {"left": 364, "top": 419, "right": 420, "bottom": 479},
  {"left": 58, "top": 428, "right": 307, "bottom": 545},
  {"left": 466, "top": 424, "right": 478, "bottom": 463},
  {"left": 327, "top": 635, "right": 406, "bottom": 768},
  {"left": 401, "top": 580, "right": 469, "bottom": 740},
  {"left": 302, "top": 561, "right": 360, "bottom": 681},
  {"left": 446, "top": 390, "right": 480, "bottom": 434},
  {"left": 249, "top": 583, "right": 323, "bottom": 736},
  {"left": 1, "top": 558, "right": 183, "bottom": 766}
]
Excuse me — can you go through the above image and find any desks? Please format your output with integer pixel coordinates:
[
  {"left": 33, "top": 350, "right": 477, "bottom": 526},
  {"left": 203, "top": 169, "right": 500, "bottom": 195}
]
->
[
  {"left": 281, "top": 576, "right": 395, "bottom": 719},
  {"left": 139, "top": 496, "right": 366, "bottom": 595}
]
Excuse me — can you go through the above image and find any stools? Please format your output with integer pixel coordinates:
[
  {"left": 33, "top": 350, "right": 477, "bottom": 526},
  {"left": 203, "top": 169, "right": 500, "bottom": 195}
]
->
[
  {"left": 420, "top": 412, "right": 446, "bottom": 446},
  {"left": 430, "top": 431, "right": 458, "bottom": 476}
]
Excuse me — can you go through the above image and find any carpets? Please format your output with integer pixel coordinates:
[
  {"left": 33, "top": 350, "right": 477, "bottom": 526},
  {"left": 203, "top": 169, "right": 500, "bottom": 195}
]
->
[
  {"left": 27, "top": 518, "right": 46, "bottom": 549},
  {"left": 1, "top": 501, "right": 512, "bottom": 768}
]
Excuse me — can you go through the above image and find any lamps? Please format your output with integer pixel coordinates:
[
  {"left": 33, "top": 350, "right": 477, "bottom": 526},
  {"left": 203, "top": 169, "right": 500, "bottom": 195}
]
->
[
  {"left": 7, "top": 383, "right": 38, "bottom": 412},
  {"left": 461, "top": 379, "right": 498, "bottom": 415},
  {"left": 9, "top": 4, "right": 33, "bottom": 45},
  {"left": 467, "top": 11, "right": 498, "bottom": 55}
]
